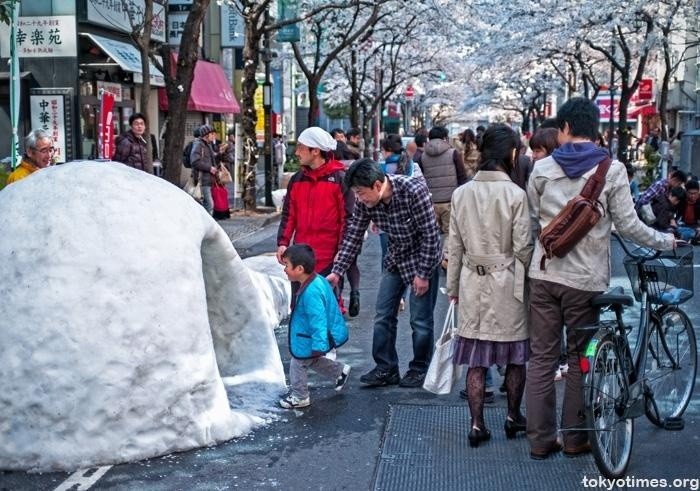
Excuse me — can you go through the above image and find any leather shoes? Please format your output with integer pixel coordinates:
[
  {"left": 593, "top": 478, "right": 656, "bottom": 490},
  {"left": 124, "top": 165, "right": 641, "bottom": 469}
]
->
[
  {"left": 564, "top": 442, "right": 596, "bottom": 457},
  {"left": 529, "top": 437, "right": 563, "bottom": 461}
]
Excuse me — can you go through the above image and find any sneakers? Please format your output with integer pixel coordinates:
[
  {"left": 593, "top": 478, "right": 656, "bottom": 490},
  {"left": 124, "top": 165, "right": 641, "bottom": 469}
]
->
[
  {"left": 334, "top": 364, "right": 351, "bottom": 392},
  {"left": 399, "top": 369, "right": 427, "bottom": 388},
  {"left": 360, "top": 366, "right": 400, "bottom": 386},
  {"left": 280, "top": 394, "right": 311, "bottom": 409}
]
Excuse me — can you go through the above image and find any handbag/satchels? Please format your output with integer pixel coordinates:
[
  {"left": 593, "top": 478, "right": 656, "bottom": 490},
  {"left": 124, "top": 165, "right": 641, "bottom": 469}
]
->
[
  {"left": 423, "top": 298, "right": 461, "bottom": 395},
  {"left": 214, "top": 161, "right": 233, "bottom": 184},
  {"left": 181, "top": 176, "right": 204, "bottom": 203},
  {"left": 212, "top": 177, "right": 230, "bottom": 219}
]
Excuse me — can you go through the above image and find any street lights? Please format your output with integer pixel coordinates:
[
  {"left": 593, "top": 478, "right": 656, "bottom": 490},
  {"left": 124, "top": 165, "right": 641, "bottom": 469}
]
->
[{"left": 258, "top": 4, "right": 278, "bottom": 209}]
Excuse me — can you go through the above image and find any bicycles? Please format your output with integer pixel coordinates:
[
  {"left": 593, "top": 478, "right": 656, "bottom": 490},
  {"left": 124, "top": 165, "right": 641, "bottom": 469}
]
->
[{"left": 558, "top": 231, "right": 698, "bottom": 479}]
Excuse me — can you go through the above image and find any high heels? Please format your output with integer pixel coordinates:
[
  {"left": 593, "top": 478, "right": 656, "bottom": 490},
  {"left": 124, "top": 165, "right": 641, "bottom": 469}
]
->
[
  {"left": 468, "top": 430, "right": 492, "bottom": 448},
  {"left": 350, "top": 290, "right": 360, "bottom": 317},
  {"left": 504, "top": 416, "right": 528, "bottom": 439}
]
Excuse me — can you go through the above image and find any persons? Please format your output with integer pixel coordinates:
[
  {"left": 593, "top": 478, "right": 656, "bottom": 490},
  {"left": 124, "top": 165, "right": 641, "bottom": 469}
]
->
[
  {"left": 182, "top": 96, "right": 700, "bottom": 460},
  {"left": 111, "top": 112, "right": 149, "bottom": 172},
  {"left": 7, "top": 129, "right": 54, "bottom": 186}
]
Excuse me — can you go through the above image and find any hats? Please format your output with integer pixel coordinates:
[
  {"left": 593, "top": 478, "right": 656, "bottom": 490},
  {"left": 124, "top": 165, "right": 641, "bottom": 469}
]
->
[
  {"left": 199, "top": 124, "right": 216, "bottom": 137},
  {"left": 298, "top": 127, "right": 337, "bottom": 151}
]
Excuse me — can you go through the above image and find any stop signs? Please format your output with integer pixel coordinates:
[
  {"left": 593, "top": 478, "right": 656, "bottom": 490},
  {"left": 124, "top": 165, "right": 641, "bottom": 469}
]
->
[{"left": 404, "top": 87, "right": 416, "bottom": 97}]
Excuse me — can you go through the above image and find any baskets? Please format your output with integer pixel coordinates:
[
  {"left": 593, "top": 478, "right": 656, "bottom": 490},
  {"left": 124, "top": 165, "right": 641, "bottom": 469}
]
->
[{"left": 623, "top": 241, "right": 695, "bottom": 305}]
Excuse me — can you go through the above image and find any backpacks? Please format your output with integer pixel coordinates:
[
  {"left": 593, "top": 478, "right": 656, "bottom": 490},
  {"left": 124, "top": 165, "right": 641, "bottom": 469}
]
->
[
  {"left": 183, "top": 141, "right": 192, "bottom": 169},
  {"left": 539, "top": 195, "right": 602, "bottom": 259}
]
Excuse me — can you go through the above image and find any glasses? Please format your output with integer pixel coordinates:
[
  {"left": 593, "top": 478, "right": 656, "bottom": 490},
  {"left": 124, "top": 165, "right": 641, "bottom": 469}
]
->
[{"left": 31, "top": 147, "right": 55, "bottom": 154}]
